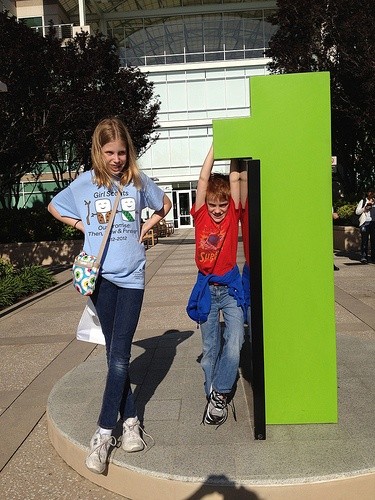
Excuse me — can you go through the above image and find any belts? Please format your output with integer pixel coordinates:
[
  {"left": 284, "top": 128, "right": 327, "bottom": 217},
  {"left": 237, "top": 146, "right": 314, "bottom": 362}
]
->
[{"left": 209, "top": 282, "right": 228, "bottom": 287}]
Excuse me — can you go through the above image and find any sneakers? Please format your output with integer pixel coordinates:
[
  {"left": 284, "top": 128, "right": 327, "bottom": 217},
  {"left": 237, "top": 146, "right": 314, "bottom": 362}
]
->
[
  {"left": 119, "top": 416, "right": 155, "bottom": 453},
  {"left": 85, "top": 428, "right": 116, "bottom": 474},
  {"left": 200, "top": 390, "right": 237, "bottom": 430}
]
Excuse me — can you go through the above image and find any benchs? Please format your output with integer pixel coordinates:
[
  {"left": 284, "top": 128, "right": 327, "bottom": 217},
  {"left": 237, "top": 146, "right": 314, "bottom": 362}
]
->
[{"left": 142, "top": 219, "right": 175, "bottom": 252}]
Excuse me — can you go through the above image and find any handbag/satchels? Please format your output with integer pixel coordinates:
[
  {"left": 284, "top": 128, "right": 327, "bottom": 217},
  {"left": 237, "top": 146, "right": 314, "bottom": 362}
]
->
[{"left": 72, "top": 250, "right": 102, "bottom": 296}]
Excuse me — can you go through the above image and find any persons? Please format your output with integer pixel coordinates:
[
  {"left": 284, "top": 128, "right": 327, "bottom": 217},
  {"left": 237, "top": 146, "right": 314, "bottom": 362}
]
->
[
  {"left": 356, "top": 186, "right": 375, "bottom": 263},
  {"left": 332, "top": 211, "right": 340, "bottom": 272},
  {"left": 46, "top": 117, "right": 171, "bottom": 475},
  {"left": 190, "top": 142, "right": 244, "bottom": 426}
]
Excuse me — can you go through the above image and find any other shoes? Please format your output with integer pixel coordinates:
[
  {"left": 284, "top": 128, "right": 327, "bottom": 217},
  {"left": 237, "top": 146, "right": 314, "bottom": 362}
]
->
[
  {"left": 361, "top": 257, "right": 368, "bottom": 262},
  {"left": 334, "top": 265, "right": 340, "bottom": 271}
]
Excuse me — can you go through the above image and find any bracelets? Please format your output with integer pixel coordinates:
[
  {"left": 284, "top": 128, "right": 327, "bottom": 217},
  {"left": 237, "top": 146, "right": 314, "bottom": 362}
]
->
[{"left": 74, "top": 220, "right": 81, "bottom": 229}]
